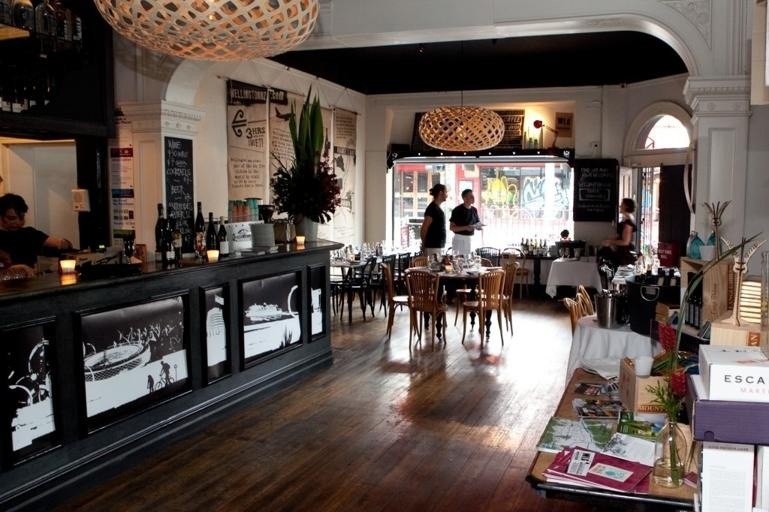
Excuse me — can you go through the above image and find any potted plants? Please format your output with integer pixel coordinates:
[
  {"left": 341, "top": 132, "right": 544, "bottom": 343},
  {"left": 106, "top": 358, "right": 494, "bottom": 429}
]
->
[{"left": 269, "top": 83, "right": 342, "bottom": 244}]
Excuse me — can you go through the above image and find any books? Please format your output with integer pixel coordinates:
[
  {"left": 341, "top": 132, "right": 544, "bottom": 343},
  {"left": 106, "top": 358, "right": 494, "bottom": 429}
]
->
[{"left": 536, "top": 383, "right": 666, "bottom": 495}]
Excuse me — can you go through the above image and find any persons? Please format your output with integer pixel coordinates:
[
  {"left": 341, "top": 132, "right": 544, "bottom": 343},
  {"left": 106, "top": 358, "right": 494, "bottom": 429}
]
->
[
  {"left": 421, "top": 183, "right": 448, "bottom": 263},
  {"left": 450, "top": 189, "right": 480, "bottom": 255},
  {"left": 0, "top": 193, "right": 73, "bottom": 275},
  {"left": 603, "top": 198, "right": 636, "bottom": 265}
]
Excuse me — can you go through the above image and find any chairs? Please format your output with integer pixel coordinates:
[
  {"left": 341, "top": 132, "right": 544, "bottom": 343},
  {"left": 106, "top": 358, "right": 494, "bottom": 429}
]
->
[
  {"left": 329, "top": 246, "right": 530, "bottom": 347},
  {"left": 563, "top": 284, "right": 594, "bottom": 334},
  {"left": 625, "top": 280, "right": 680, "bottom": 337}
]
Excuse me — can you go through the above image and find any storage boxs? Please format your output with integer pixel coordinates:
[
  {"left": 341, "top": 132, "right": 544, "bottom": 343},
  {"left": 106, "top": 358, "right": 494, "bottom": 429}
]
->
[
  {"left": 620, "top": 359, "right": 685, "bottom": 418},
  {"left": 686, "top": 374, "right": 769, "bottom": 445},
  {"left": 698, "top": 344, "right": 769, "bottom": 403},
  {"left": 693, "top": 440, "right": 769, "bottom": 512}
]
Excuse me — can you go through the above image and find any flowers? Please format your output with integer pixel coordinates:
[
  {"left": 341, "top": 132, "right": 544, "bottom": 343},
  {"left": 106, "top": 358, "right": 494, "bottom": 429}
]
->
[{"left": 645, "top": 231, "right": 762, "bottom": 421}]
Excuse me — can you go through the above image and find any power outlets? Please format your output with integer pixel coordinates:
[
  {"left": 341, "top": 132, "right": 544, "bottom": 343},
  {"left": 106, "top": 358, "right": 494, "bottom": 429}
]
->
[{"left": 592, "top": 143, "right": 600, "bottom": 151}]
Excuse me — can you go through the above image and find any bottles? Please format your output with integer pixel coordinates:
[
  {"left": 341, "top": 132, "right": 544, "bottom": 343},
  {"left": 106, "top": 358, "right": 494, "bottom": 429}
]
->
[
  {"left": 153, "top": 202, "right": 230, "bottom": 270},
  {"left": 520, "top": 237, "right": 547, "bottom": 256},
  {"left": 330, "top": 240, "right": 386, "bottom": 264},
  {"left": 685, "top": 272, "right": 702, "bottom": 330}
]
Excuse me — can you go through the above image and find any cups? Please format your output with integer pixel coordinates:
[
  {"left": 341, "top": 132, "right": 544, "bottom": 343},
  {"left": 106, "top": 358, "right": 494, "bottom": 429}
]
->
[
  {"left": 60, "top": 274, "right": 77, "bottom": 285},
  {"left": 60, "top": 260, "right": 76, "bottom": 273},
  {"left": 699, "top": 246, "right": 715, "bottom": 261},
  {"left": 296, "top": 236, "right": 305, "bottom": 244},
  {"left": 573, "top": 248, "right": 580, "bottom": 257},
  {"left": 427, "top": 249, "right": 481, "bottom": 273}
]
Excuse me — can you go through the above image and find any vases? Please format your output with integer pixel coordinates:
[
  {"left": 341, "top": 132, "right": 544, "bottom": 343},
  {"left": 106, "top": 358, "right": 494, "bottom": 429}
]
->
[{"left": 655, "top": 417, "right": 688, "bottom": 489}]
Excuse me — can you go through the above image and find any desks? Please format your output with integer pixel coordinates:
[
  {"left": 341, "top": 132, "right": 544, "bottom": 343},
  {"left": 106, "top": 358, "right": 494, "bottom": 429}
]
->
[
  {"left": 525, "top": 367, "right": 695, "bottom": 512},
  {"left": 611, "top": 264, "right": 680, "bottom": 292}
]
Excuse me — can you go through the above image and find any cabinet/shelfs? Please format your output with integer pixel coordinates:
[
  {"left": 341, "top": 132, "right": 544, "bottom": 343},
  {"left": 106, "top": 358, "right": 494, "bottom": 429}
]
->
[
  {"left": 1, "top": 0, "right": 117, "bottom": 138},
  {"left": 495, "top": 255, "right": 554, "bottom": 301}
]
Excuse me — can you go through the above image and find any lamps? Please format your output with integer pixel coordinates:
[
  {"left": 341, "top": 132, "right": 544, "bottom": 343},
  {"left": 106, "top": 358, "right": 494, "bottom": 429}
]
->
[
  {"left": 534, "top": 120, "right": 560, "bottom": 135},
  {"left": 93, "top": 0, "right": 321, "bottom": 62},
  {"left": 418, "top": 91, "right": 506, "bottom": 152}
]
